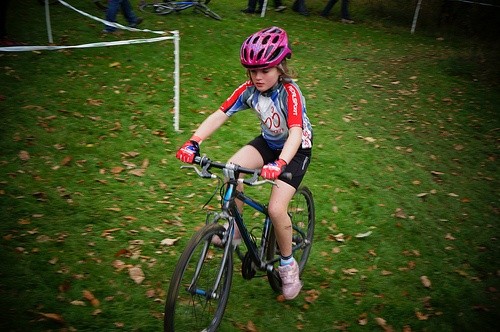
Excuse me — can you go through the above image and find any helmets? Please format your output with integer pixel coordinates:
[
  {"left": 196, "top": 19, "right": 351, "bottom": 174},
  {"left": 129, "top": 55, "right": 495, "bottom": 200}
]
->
[{"left": 240, "top": 25, "right": 292, "bottom": 70}]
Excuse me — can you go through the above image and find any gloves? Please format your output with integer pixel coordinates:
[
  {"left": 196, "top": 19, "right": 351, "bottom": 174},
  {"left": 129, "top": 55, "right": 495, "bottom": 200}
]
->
[
  {"left": 175, "top": 135, "right": 202, "bottom": 164},
  {"left": 260, "top": 159, "right": 287, "bottom": 181}
]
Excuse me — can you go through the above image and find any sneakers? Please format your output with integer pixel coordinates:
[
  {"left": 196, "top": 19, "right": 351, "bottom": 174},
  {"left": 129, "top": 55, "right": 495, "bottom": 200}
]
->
[
  {"left": 278, "top": 256, "right": 302, "bottom": 300},
  {"left": 274, "top": 5, "right": 287, "bottom": 12},
  {"left": 211, "top": 221, "right": 241, "bottom": 245}
]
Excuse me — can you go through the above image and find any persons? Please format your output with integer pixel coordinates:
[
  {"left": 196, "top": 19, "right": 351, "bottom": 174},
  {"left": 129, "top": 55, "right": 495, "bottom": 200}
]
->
[
  {"left": 175, "top": 26, "right": 313, "bottom": 301},
  {"left": 241, "top": 0, "right": 264, "bottom": 14},
  {"left": 105, "top": 0, "right": 145, "bottom": 33},
  {"left": 292, "top": 0, "right": 309, "bottom": 16},
  {"left": 273, "top": 0, "right": 287, "bottom": 12},
  {"left": 320, "top": 0, "right": 355, "bottom": 24}
]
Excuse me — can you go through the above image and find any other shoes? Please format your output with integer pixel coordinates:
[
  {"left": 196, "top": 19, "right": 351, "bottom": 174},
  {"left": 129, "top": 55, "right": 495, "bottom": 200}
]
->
[
  {"left": 128, "top": 16, "right": 144, "bottom": 27},
  {"left": 103, "top": 29, "right": 124, "bottom": 35},
  {"left": 240, "top": 7, "right": 262, "bottom": 14},
  {"left": 341, "top": 18, "right": 355, "bottom": 24}
]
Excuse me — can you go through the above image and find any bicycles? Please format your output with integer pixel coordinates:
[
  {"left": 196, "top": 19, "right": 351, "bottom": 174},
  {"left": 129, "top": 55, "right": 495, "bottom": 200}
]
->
[
  {"left": 164, "top": 146, "right": 316, "bottom": 332},
  {"left": 138, "top": 0, "right": 221, "bottom": 25}
]
